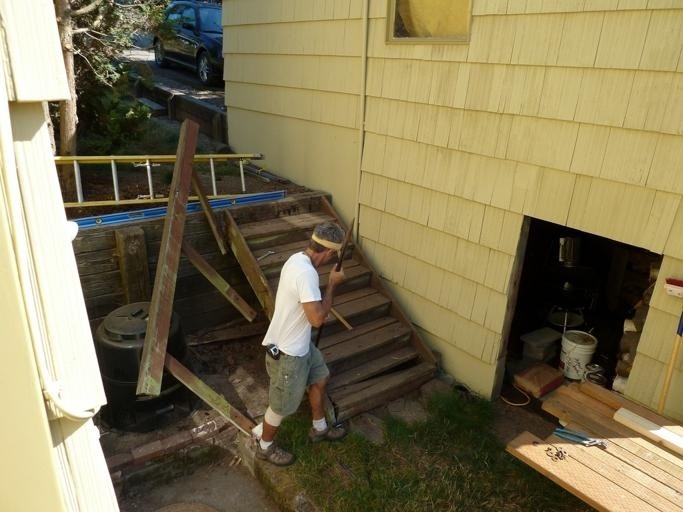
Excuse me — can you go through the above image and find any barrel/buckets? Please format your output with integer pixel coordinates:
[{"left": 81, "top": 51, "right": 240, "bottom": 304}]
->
[{"left": 560, "top": 328, "right": 599, "bottom": 380}]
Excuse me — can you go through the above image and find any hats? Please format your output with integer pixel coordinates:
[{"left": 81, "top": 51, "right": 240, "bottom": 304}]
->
[{"left": 311, "top": 233, "right": 343, "bottom": 261}]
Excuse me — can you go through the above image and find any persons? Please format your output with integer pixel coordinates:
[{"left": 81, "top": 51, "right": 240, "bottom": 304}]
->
[{"left": 254, "top": 220, "right": 348, "bottom": 467}]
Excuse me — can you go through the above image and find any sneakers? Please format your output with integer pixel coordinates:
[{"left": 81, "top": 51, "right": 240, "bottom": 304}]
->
[
  {"left": 307, "top": 423, "right": 345, "bottom": 442},
  {"left": 256, "top": 441, "right": 294, "bottom": 465}
]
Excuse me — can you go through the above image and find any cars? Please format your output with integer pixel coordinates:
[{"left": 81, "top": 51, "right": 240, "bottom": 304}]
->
[{"left": 153, "top": 0, "right": 223, "bottom": 86}]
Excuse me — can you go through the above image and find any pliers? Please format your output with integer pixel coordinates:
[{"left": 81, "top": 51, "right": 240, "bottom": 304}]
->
[{"left": 553, "top": 428, "right": 608, "bottom": 448}]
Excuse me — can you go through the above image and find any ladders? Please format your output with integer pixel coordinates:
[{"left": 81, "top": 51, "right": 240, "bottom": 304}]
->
[{"left": 54, "top": 154, "right": 265, "bottom": 208}]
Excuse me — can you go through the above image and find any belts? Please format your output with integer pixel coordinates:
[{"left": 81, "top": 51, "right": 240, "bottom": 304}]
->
[{"left": 279, "top": 350, "right": 291, "bottom": 357}]
